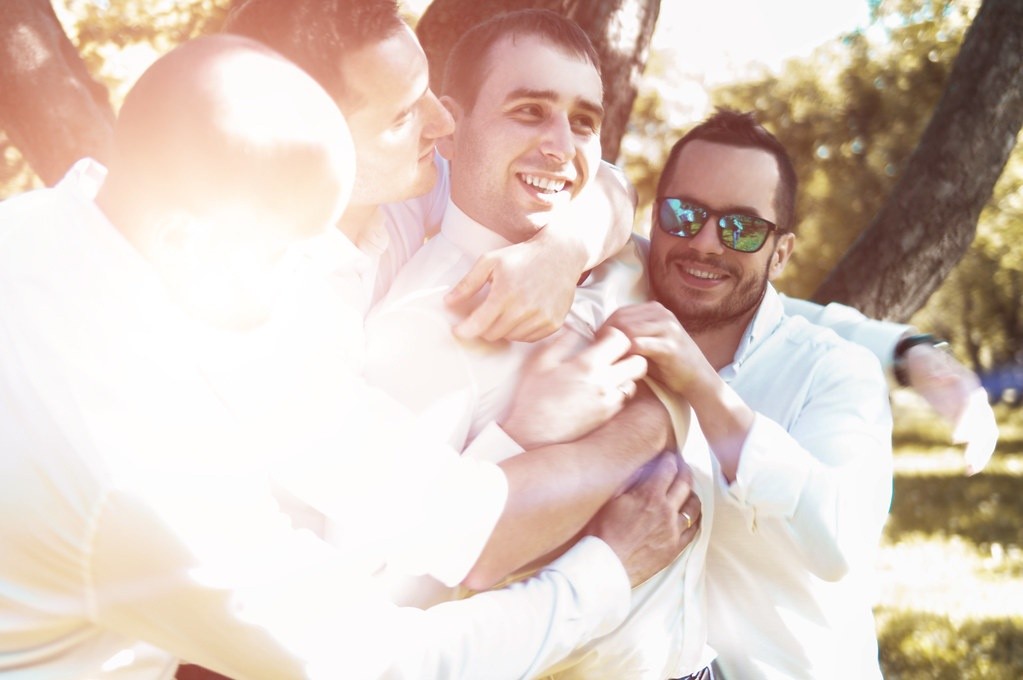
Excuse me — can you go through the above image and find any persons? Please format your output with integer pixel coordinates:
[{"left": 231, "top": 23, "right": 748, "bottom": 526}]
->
[
  {"left": 731, "top": 218, "right": 742, "bottom": 249},
  {"left": 702, "top": 212, "right": 706, "bottom": 219},
  {"left": 679, "top": 205, "right": 694, "bottom": 234},
  {"left": 0, "top": 0, "right": 999, "bottom": 679}
]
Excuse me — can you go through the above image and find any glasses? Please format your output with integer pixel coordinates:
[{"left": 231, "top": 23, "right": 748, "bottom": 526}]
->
[{"left": 656, "top": 196, "right": 784, "bottom": 253}]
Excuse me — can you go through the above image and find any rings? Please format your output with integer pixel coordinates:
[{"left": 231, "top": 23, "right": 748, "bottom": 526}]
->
[
  {"left": 682, "top": 512, "right": 691, "bottom": 527},
  {"left": 619, "top": 388, "right": 629, "bottom": 400}
]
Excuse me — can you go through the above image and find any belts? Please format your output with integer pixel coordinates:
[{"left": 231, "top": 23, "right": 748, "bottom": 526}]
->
[{"left": 670, "top": 660, "right": 722, "bottom": 680}]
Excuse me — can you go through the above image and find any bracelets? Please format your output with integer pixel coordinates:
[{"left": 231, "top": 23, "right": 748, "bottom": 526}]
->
[{"left": 896, "top": 334, "right": 951, "bottom": 386}]
